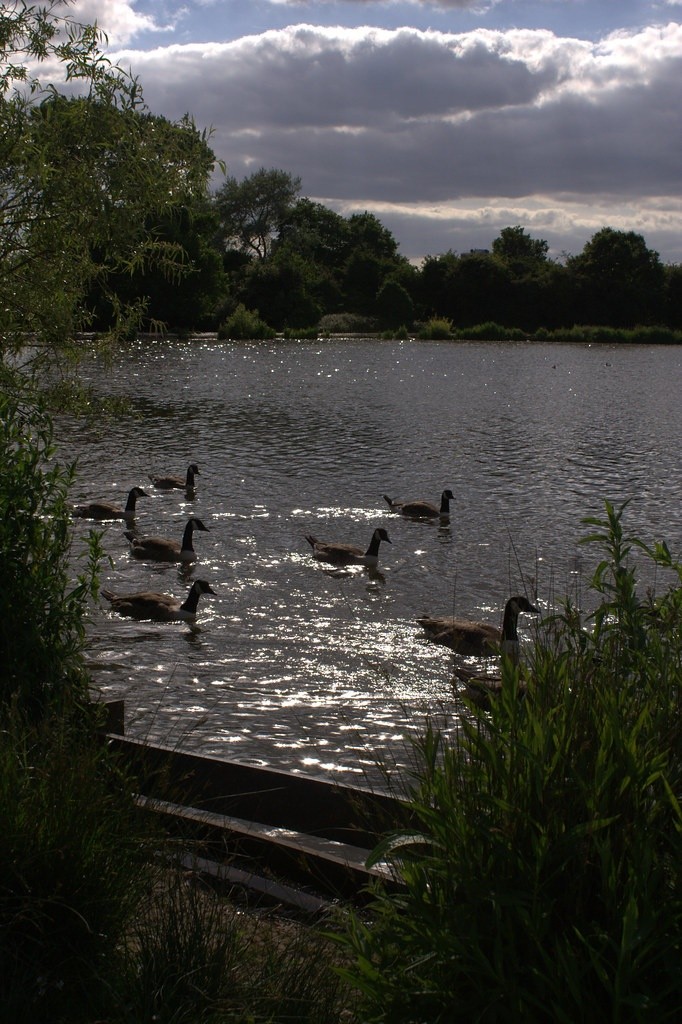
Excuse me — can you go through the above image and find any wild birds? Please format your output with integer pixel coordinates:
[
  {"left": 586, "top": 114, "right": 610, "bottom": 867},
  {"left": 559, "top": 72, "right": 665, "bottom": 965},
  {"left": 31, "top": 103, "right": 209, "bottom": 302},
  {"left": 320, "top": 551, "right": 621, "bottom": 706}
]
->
[
  {"left": 123, "top": 517, "right": 211, "bottom": 564},
  {"left": 304, "top": 528, "right": 393, "bottom": 568},
  {"left": 100, "top": 579, "right": 218, "bottom": 624},
  {"left": 148, "top": 464, "right": 202, "bottom": 490},
  {"left": 71, "top": 487, "right": 150, "bottom": 522},
  {"left": 414, "top": 595, "right": 541, "bottom": 656},
  {"left": 381, "top": 490, "right": 456, "bottom": 521}
]
